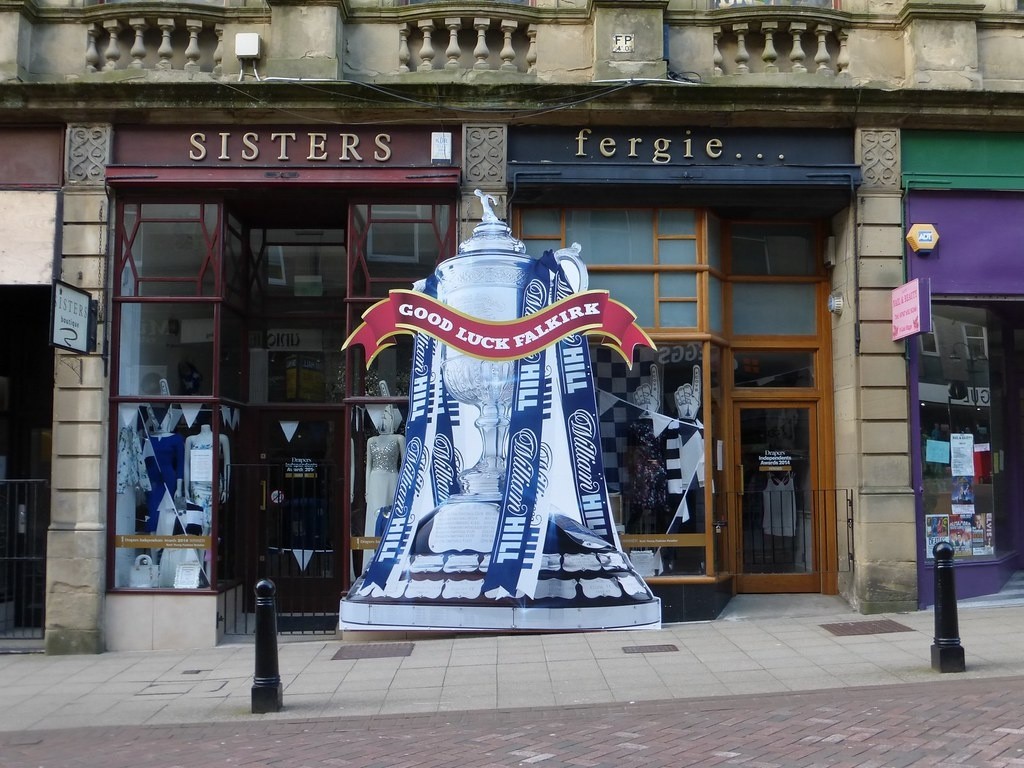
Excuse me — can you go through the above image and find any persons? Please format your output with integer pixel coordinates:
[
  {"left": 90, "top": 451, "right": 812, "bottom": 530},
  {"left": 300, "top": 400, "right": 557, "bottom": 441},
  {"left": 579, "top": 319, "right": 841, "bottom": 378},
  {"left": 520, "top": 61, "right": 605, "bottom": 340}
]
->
[
  {"left": 361, "top": 405, "right": 405, "bottom": 577},
  {"left": 927, "top": 514, "right": 985, "bottom": 551},
  {"left": 142, "top": 424, "right": 231, "bottom": 587}
]
